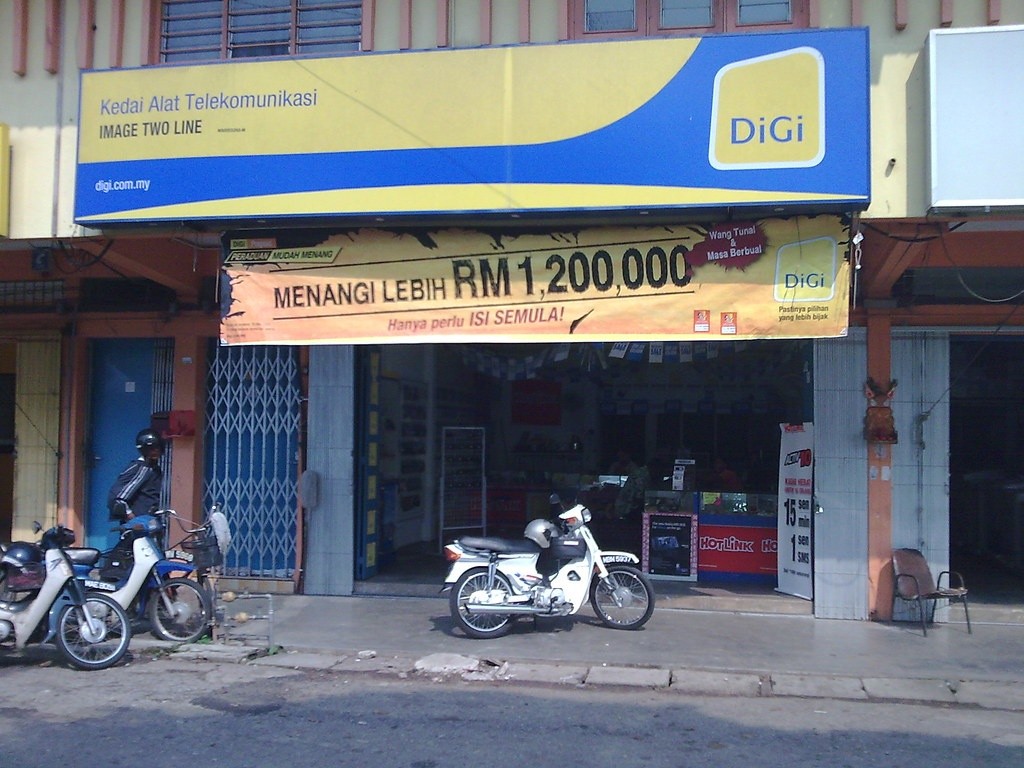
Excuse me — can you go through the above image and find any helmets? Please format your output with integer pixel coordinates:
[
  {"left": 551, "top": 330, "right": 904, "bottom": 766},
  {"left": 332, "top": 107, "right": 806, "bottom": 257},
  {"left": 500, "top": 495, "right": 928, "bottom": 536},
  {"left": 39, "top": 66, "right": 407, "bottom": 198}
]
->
[
  {"left": 523, "top": 518, "right": 559, "bottom": 548},
  {"left": 1, "top": 541, "right": 41, "bottom": 570},
  {"left": 135, "top": 429, "right": 163, "bottom": 453}
]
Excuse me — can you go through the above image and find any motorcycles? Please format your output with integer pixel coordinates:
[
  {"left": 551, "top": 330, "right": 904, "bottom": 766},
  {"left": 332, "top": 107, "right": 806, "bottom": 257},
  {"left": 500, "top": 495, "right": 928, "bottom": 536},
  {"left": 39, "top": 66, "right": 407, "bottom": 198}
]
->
[
  {"left": 44, "top": 511, "right": 214, "bottom": 643},
  {"left": 0, "top": 520, "right": 132, "bottom": 672},
  {"left": 437, "top": 480, "right": 656, "bottom": 640}
]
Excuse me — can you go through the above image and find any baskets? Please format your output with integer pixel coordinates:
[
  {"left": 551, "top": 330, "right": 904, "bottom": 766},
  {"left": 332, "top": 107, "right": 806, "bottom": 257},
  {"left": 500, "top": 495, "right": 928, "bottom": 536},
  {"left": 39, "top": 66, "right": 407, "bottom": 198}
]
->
[{"left": 178, "top": 532, "right": 225, "bottom": 569}]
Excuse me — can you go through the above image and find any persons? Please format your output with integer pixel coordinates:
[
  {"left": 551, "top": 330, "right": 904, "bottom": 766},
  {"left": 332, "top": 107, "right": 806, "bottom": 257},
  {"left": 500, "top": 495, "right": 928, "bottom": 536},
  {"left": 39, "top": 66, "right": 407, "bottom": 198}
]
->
[
  {"left": 709, "top": 457, "right": 739, "bottom": 489},
  {"left": 107, "top": 430, "right": 170, "bottom": 619}
]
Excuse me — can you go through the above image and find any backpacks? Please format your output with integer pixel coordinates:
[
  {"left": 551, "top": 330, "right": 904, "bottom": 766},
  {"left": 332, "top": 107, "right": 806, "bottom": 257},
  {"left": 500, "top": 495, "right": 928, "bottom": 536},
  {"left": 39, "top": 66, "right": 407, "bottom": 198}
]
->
[{"left": 98, "top": 530, "right": 148, "bottom": 584}]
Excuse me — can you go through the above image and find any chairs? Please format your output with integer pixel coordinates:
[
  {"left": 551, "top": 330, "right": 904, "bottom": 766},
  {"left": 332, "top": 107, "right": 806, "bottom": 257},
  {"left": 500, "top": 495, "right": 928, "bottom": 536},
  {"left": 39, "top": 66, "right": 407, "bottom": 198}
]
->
[{"left": 888, "top": 548, "right": 973, "bottom": 639}]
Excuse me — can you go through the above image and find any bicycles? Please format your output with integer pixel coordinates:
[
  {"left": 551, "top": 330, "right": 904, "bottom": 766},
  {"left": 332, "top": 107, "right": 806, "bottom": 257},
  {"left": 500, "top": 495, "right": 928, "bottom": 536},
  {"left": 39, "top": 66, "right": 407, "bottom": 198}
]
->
[{"left": 101, "top": 502, "right": 224, "bottom": 612}]
